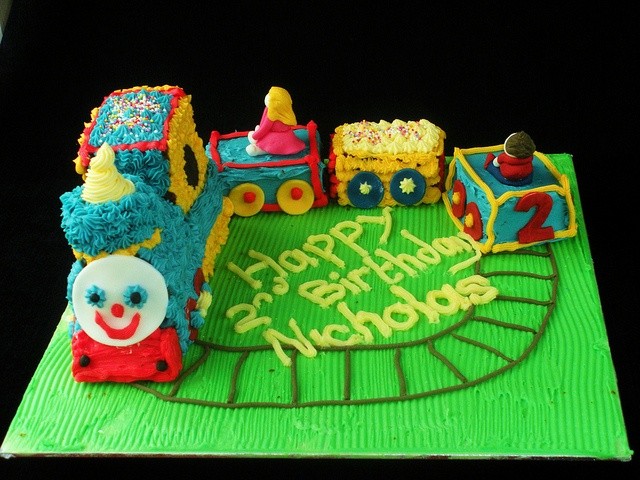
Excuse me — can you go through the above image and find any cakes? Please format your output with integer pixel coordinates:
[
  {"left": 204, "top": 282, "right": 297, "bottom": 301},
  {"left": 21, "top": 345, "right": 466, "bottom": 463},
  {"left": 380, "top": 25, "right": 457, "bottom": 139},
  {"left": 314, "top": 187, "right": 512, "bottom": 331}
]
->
[{"left": 1, "top": 85, "right": 636, "bottom": 463}]
